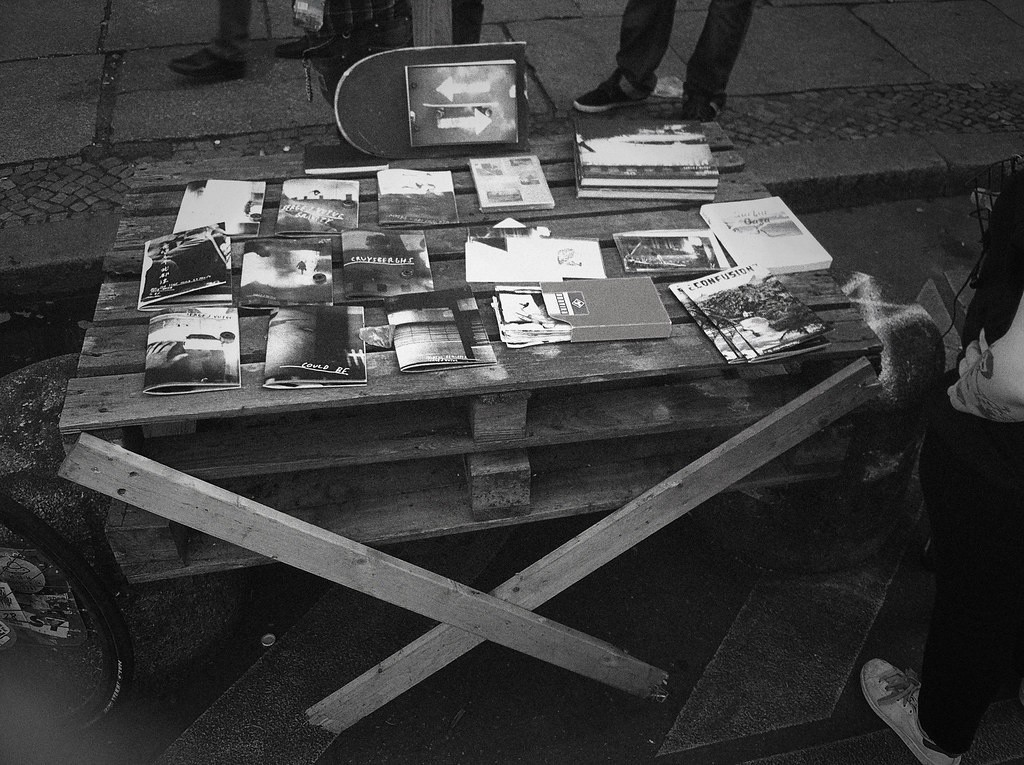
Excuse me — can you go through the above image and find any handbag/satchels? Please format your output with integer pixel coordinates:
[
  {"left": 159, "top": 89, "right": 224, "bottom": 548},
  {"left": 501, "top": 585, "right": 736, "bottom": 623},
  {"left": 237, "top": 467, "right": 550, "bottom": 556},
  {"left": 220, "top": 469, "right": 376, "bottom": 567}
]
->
[{"left": 301, "top": 16, "right": 415, "bottom": 111}]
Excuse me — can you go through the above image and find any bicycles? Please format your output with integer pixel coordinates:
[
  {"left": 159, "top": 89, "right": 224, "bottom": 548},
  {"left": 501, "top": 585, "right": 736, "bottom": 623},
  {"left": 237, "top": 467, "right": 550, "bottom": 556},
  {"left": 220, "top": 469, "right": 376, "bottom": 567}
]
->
[{"left": 0, "top": 491, "right": 136, "bottom": 765}]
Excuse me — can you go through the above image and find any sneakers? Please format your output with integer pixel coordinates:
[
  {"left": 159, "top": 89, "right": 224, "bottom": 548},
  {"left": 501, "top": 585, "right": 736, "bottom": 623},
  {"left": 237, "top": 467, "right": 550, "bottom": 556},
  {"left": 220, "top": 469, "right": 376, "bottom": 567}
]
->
[
  {"left": 573, "top": 75, "right": 647, "bottom": 112},
  {"left": 861, "top": 658, "right": 962, "bottom": 764},
  {"left": 678, "top": 94, "right": 724, "bottom": 121}
]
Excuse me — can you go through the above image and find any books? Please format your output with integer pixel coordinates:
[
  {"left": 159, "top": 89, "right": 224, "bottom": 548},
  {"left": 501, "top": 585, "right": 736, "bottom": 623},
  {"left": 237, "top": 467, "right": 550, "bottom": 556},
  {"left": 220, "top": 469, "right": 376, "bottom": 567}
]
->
[{"left": 138, "top": 116, "right": 852, "bottom": 396}]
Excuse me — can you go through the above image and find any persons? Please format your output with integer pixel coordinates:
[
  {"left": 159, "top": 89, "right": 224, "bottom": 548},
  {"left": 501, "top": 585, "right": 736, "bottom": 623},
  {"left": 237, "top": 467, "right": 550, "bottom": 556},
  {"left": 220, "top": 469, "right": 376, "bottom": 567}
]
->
[
  {"left": 573, "top": 0, "right": 755, "bottom": 123},
  {"left": 861, "top": 170, "right": 1024, "bottom": 765},
  {"left": 142, "top": 243, "right": 180, "bottom": 298},
  {"left": 168, "top": 0, "right": 335, "bottom": 76}
]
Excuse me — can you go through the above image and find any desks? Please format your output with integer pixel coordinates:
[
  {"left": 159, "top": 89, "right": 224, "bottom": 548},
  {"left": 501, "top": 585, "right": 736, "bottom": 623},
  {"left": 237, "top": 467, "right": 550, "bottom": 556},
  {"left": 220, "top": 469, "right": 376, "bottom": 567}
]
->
[{"left": 57, "top": 121, "right": 885, "bottom": 736}]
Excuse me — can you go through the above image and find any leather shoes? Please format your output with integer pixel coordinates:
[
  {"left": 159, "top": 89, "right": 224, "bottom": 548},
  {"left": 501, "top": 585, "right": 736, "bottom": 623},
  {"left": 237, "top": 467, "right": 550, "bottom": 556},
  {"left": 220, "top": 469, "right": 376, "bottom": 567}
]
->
[
  {"left": 168, "top": 48, "right": 248, "bottom": 79},
  {"left": 276, "top": 34, "right": 308, "bottom": 59}
]
